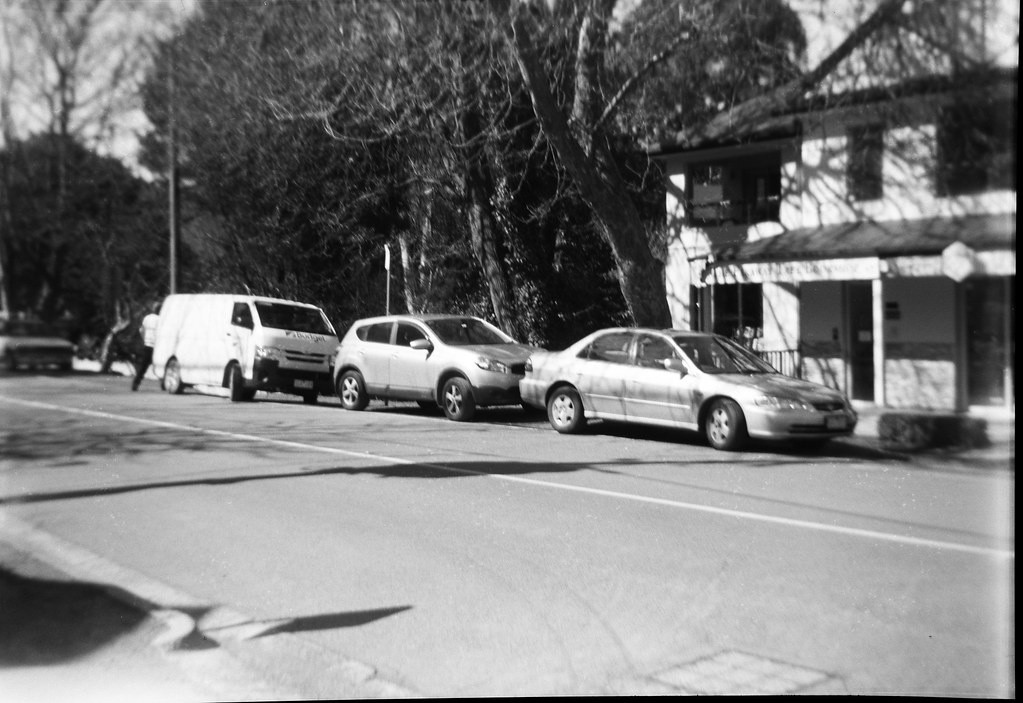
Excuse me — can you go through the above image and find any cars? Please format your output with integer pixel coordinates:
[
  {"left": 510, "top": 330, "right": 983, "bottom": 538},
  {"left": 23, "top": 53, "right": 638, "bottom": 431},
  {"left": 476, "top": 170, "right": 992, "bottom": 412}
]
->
[
  {"left": 337, "top": 314, "right": 550, "bottom": 423},
  {"left": 518, "top": 326, "right": 858, "bottom": 450},
  {"left": 0, "top": 312, "right": 78, "bottom": 374}
]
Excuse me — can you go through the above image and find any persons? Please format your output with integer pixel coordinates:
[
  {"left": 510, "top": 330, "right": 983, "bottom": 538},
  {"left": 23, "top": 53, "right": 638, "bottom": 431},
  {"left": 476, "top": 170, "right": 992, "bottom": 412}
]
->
[{"left": 130, "top": 301, "right": 166, "bottom": 391}]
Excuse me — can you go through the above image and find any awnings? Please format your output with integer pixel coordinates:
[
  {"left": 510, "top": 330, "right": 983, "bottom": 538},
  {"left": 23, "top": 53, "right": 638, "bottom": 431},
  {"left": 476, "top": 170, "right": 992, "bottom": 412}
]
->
[{"left": 699, "top": 208, "right": 1019, "bottom": 306}]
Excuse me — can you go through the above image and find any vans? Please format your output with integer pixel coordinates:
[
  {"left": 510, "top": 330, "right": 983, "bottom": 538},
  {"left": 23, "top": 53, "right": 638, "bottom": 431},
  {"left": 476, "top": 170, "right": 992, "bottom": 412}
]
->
[{"left": 153, "top": 293, "right": 341, "bottom": 406}]
